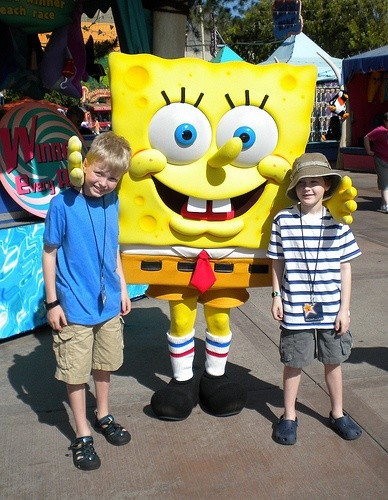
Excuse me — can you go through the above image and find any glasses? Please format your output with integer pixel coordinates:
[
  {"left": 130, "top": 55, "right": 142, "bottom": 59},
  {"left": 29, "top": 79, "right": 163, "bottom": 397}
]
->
[{"left": 65, "top": 111, "right": 80, "bottom": 120}]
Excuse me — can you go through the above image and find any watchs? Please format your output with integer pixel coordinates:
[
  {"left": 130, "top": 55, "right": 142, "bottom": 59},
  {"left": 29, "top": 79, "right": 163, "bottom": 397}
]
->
[
  {"left": 272, "top": 291, "right": 281, "bottom": 297},
  {"left": 43, "top": 300, "right": 59, "bottom": 310}
]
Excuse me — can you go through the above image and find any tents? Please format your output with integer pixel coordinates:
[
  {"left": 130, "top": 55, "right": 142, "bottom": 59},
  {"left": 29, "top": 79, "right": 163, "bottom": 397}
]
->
[
  {"left": 341, "top": 44, "right": 388, "bottom": 84},
  {"left": 209, "top": 45, "right": 243, "bottom": 64},
  {"left": 258, "top": 32, "right": 343, "bottom": 82}
]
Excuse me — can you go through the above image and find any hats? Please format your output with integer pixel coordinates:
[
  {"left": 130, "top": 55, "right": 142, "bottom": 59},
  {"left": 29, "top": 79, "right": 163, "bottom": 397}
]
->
[{"left": 286, "top": 153, "right": 341, "bottom": 202}]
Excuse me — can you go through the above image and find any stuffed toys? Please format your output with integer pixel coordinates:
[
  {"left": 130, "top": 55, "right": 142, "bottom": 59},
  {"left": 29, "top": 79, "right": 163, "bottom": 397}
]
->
[{"left": 329, "top": 89, "right": 350, "bottom": 118}]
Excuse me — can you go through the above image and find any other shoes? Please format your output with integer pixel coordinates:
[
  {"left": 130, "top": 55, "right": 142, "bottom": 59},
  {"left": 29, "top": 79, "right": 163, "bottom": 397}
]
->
[{"left": 378, "top": 205, "right": 388, "bottom": 213}]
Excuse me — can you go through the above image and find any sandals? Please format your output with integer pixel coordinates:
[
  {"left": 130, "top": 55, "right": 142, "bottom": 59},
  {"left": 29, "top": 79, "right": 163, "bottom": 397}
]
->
[
  {"left": 274, "top": 415, "right": 298, "bottom": 444},
  {"left": 94, "top": 412, "right": 131, "bottom": 445},
  {"left": 71, "top": 436, "right": 101, "bottom": 470},
  {"left": 329, "top": 411, "right": 362, "bottom": 440}
]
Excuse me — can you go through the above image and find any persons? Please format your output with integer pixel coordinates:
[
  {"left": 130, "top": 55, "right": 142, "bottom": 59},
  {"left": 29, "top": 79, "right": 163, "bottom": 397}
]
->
[
  {"left": 325, "top": 116, "right": 342, "bottom": 141},
  {"left": 69, "top": 53, "right": 358, "bottom": 422},
  {"left": 66, "top": 104, "right": 95, "bottom": 141},
  {"left": 364, "top": 113, "right": 388, "bottom": 213},
  {"left": 94, "top": 119, "right": 102, "bottom": 135},
  {"left": 266, "top": 152, "right": 362, "bottom": 445},
  {"left": 41, "top": 132, "right": 132, "bottom": 470}
]
